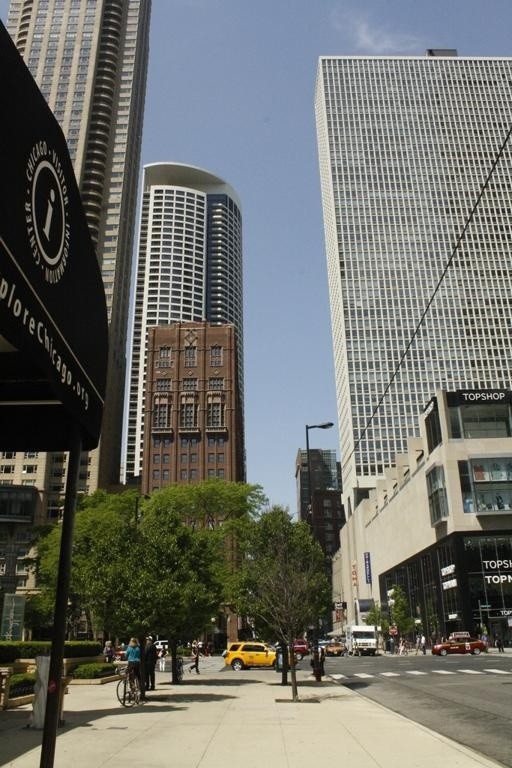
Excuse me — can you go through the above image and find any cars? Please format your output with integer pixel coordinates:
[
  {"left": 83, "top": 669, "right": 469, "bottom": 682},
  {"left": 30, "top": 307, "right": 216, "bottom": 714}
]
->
[
  {"left": 224, "top": 625, "right": 379, "bottom": 670},
  {"left": 430, "top": 630, "right": 487, "bottom": 656}
]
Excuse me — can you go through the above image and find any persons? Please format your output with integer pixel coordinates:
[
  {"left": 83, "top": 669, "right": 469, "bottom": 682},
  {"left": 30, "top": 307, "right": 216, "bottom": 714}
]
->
[
  {"left": 416, "top": 634, "right": 426, "bottom": 656},
  {"left": 399, "top": 636, "right": 409, "bottom": 657},
  {"left": 104, "top": 641, "right": 115, "bottom": 662},
  {"left": 189, "top": 640, "right": 203, "bottom": 674},
  {"left": 204, "top": 645, "right": 211, "bottom": 657},
  {"left": 494, "top": 633, "right": 505, "bottom": 652},
  {"left": 125, "top": 636, "right": 167, "bottom": 701}
]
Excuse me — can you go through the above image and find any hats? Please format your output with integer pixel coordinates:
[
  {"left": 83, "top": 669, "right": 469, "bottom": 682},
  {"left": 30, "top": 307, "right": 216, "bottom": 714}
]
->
[{"left": 146, "top": 636, "right": 153, "bottom": 640}]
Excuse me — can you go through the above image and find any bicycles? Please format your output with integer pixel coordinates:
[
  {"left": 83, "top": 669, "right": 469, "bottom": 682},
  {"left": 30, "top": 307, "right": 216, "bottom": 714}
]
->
[{"left": 114, "top": 665, "right": 147, "bottom": 708}]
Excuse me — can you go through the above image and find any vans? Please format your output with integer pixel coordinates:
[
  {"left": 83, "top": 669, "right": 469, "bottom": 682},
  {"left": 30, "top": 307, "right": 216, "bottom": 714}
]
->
[{"left": 153, "top": 640, "right": 178, "bottom": 650}]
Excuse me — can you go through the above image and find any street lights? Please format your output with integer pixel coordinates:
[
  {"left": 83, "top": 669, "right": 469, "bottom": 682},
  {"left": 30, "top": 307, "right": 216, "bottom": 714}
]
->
[{"left": 303, "top": 421, "right": 334, "bottom": 683}]
